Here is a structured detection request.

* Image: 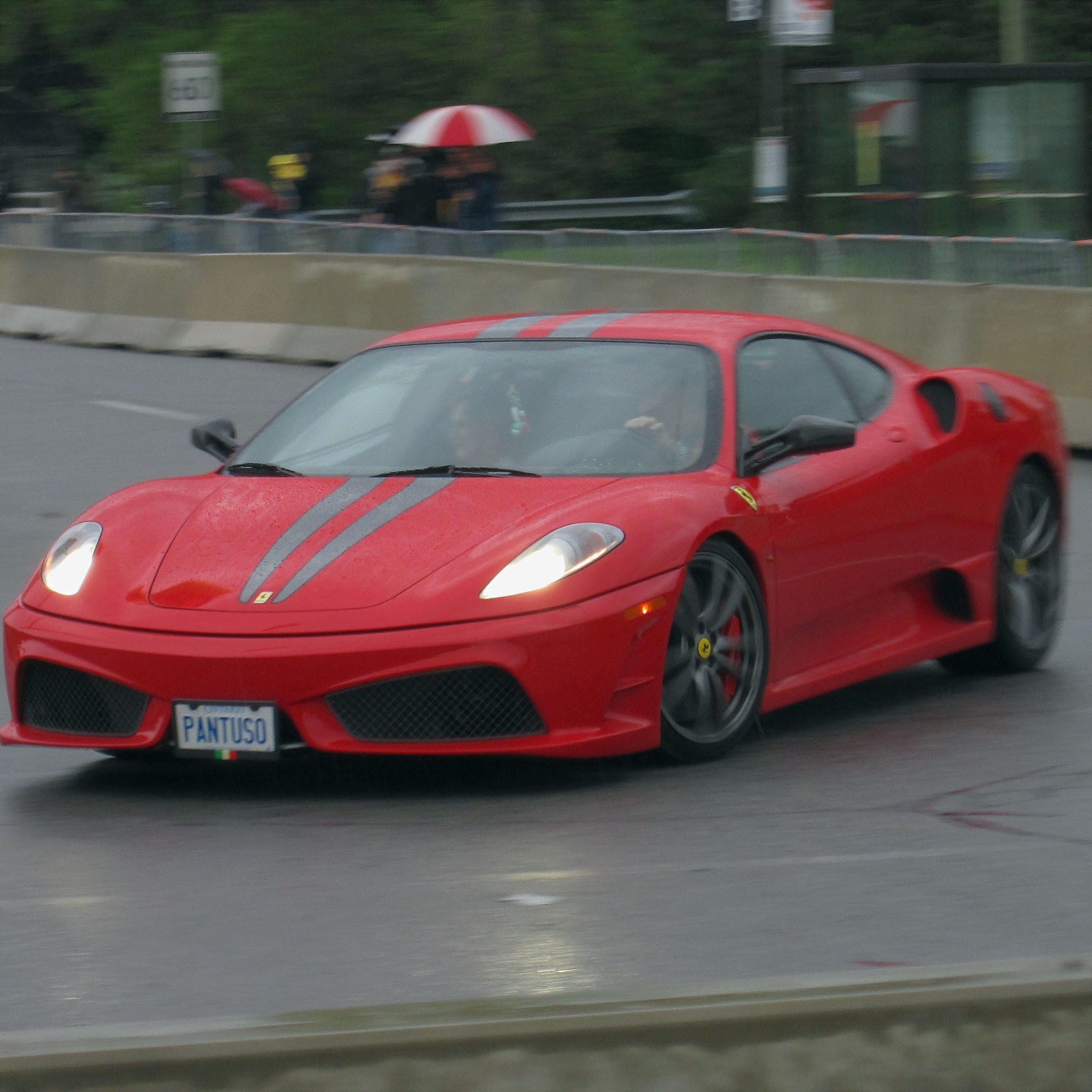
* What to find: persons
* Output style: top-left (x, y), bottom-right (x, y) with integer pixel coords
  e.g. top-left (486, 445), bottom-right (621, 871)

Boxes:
top-left (621, 342), bottom-right (761, 476)
top-left (261, 138), bottom-right (498, 234)
top-left (433, 394), bottom-right (494, 469)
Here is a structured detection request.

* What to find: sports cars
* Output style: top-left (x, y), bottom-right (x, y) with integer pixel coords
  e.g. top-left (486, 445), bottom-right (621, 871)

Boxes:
top-left (1, 309), bottom-right (1073, 772)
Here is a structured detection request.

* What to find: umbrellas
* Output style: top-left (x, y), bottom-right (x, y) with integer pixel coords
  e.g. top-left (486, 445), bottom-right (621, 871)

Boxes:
top-left (387, 104), bottom-right (535, 146)
